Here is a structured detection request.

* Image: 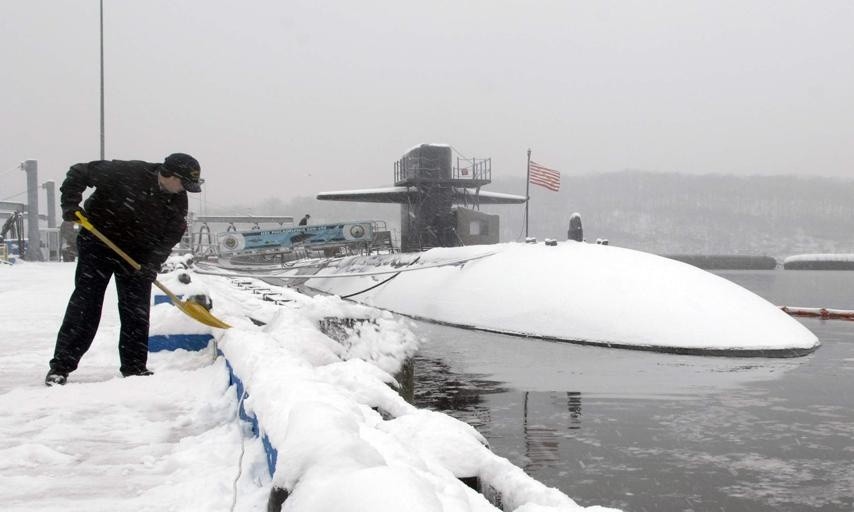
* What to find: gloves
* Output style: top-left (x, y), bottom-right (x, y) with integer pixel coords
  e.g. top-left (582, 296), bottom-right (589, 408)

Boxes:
top-left (134, 262), bottom-right (156, 283)
top-left (61, 202), bottom-right (85, 222)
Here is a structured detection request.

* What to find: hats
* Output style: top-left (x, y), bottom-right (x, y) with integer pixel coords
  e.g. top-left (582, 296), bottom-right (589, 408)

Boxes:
top-left (160, 154), bottom-right (203, 193)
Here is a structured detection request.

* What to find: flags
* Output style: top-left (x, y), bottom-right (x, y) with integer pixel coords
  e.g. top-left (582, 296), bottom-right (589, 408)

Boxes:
top-left (528, 160), bottom-right (560, 192)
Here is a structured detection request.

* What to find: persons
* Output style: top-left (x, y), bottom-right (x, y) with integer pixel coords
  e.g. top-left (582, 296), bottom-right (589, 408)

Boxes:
top-left (43, 152), bottom-right (202, 386)
top-left (298, 214), bottom-right (311, 231)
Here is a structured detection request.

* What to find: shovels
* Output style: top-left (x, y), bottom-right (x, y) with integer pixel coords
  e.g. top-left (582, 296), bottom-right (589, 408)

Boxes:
top-left (72, 212), bottom-right (232, 329)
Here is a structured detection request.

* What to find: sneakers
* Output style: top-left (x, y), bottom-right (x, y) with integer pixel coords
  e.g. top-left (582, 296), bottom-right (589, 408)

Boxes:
top-left (123, 368), bottom-right (153, 378)
top-left (44, 368), bottom-right (67, 385)
